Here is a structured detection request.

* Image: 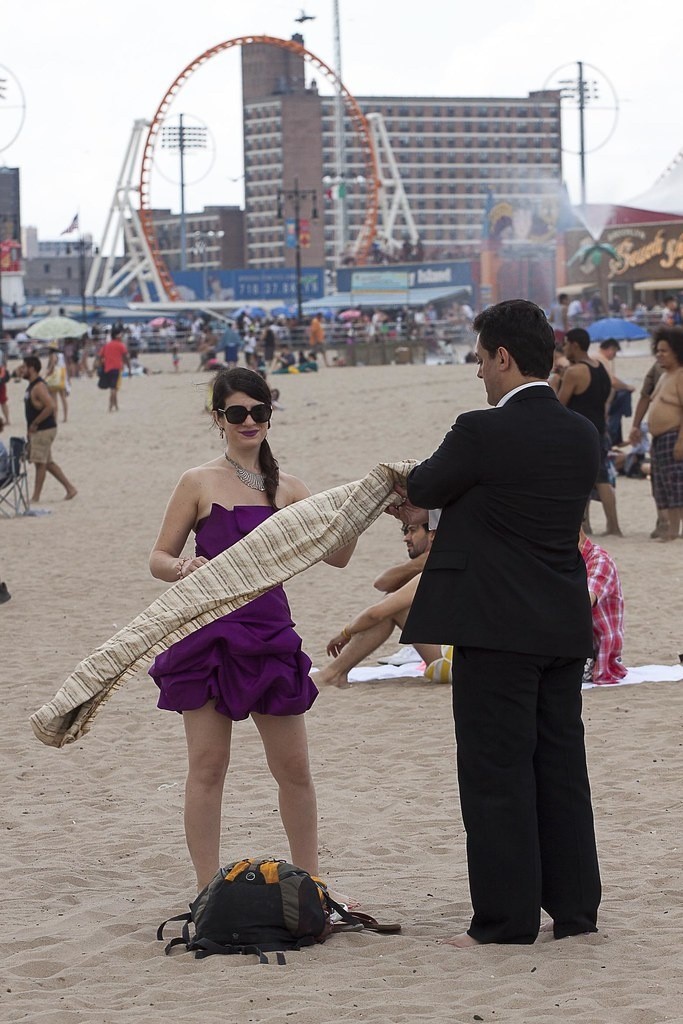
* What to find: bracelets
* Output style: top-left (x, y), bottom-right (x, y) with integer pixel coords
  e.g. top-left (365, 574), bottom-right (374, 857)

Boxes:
top-left (174, 556), bottom-right (189, 580)
top-left (341, 627), bottom-right (351, 639)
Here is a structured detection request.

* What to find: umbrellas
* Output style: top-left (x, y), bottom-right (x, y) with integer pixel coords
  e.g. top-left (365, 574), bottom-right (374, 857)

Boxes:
top-left (586, 317), bottom-right (650, 341)
top-left (26, 314), bottom-right (90, 340)
top-left (147, 317), bottom-right (175, 328)
top-left (230, 304), bottom-right (361, 322)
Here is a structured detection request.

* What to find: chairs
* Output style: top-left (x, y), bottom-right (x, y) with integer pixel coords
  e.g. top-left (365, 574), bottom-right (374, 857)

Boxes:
top-left (0, 437), bottom-right (33, 520)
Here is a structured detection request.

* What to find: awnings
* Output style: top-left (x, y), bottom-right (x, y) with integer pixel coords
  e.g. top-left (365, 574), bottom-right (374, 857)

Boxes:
top-left (294, 285), bottom-right (467, 307)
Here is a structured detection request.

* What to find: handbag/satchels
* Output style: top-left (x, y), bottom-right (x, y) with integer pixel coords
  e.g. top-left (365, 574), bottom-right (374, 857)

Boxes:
top-left (44, 365), bottom-right (64, 392)
top-left (97, 364), bottom-right (108, 389)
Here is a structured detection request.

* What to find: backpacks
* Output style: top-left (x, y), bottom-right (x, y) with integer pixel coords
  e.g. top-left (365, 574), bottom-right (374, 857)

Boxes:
top-left (156, 858), bottom-right (400, 962)
top-left (621, 450), bottom-right (647, 479)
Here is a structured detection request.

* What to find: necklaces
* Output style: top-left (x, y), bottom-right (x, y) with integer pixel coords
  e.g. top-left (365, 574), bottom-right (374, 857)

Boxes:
top-left (225, 452), bottom-right (266, 492)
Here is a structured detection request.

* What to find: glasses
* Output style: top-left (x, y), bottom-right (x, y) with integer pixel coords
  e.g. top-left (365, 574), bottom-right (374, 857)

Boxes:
top-left (218, 401), bottom-right (273, 425)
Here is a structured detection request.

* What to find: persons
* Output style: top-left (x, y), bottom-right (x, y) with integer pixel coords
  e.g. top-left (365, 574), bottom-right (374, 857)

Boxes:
top-left (11, 312), bottom-right (154, 380)
top-left (0, 328), bottom-right (133, 504)
top-left (309, 328), bottom-right (683, 689)
top-left (148, 367), bottom-right (359, 895)
top-left (548, 292), bottom-right (683, 339)
top-left (384, 298), bottom-right (602, 948)
top-left (171, 311), bottom-right (333, 374)
top-left (364, 303), bottom-right (438, 340)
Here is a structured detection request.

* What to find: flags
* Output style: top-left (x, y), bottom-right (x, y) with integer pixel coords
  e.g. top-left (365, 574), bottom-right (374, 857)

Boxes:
top-left (325, 181), bottom-right (345, 200)
top-left (59, 215), bottom-right (79, 235)
